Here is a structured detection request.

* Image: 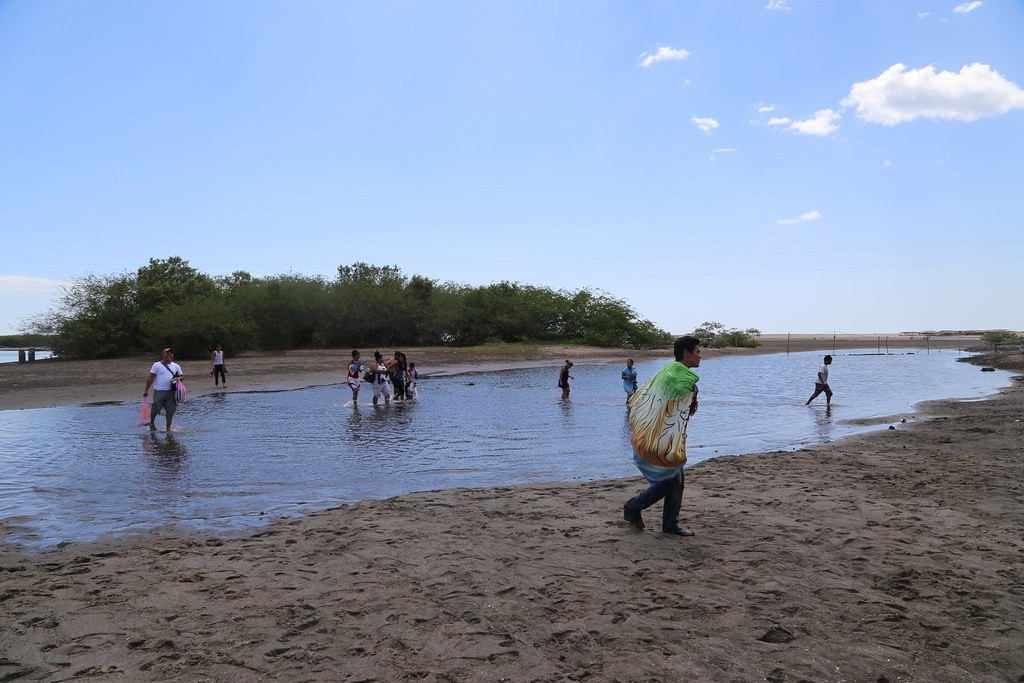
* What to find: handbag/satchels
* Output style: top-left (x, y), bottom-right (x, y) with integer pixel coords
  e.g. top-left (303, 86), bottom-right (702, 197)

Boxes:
top-left (137, 397), bottom-right (151, 427)
top-left (173, 378), bottom-right (186, 405)
top-left (404, 379), bottom-right (413, 398)
top-left (363, 371), bottom-right (375, 383)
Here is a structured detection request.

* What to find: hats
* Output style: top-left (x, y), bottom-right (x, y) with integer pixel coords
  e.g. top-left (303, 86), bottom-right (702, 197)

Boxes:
top-left (162, 348), bottom-right (174, 353)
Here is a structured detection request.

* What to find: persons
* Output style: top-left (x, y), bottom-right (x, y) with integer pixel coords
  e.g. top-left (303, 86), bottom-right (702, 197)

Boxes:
top-left (805, 355), bottom-right (833, 405)
top-left (143, 348), bottom-right (184, 431)
top-left (371, 351), bottom-right (418, 404)
top-left (211, 345), bottom-right (227, 388)
top-left (624, 336), bottom-right (702, 536)
top-left (622, 359), bottom-right (637, 404)
top-left (558, 360), bottom-right (574, 399)
top-left (347, 350), bottom-right (363, 408)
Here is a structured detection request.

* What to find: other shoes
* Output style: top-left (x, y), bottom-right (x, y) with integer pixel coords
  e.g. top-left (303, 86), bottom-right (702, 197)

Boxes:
top-left (215, 385), bottom-right (221, 389)
top-left (623, 511), bottom-right (645, 529)
top-left (223, 385), bottom-right (228, 388)
top-left (663, 526), bottom-right (694, 536)
